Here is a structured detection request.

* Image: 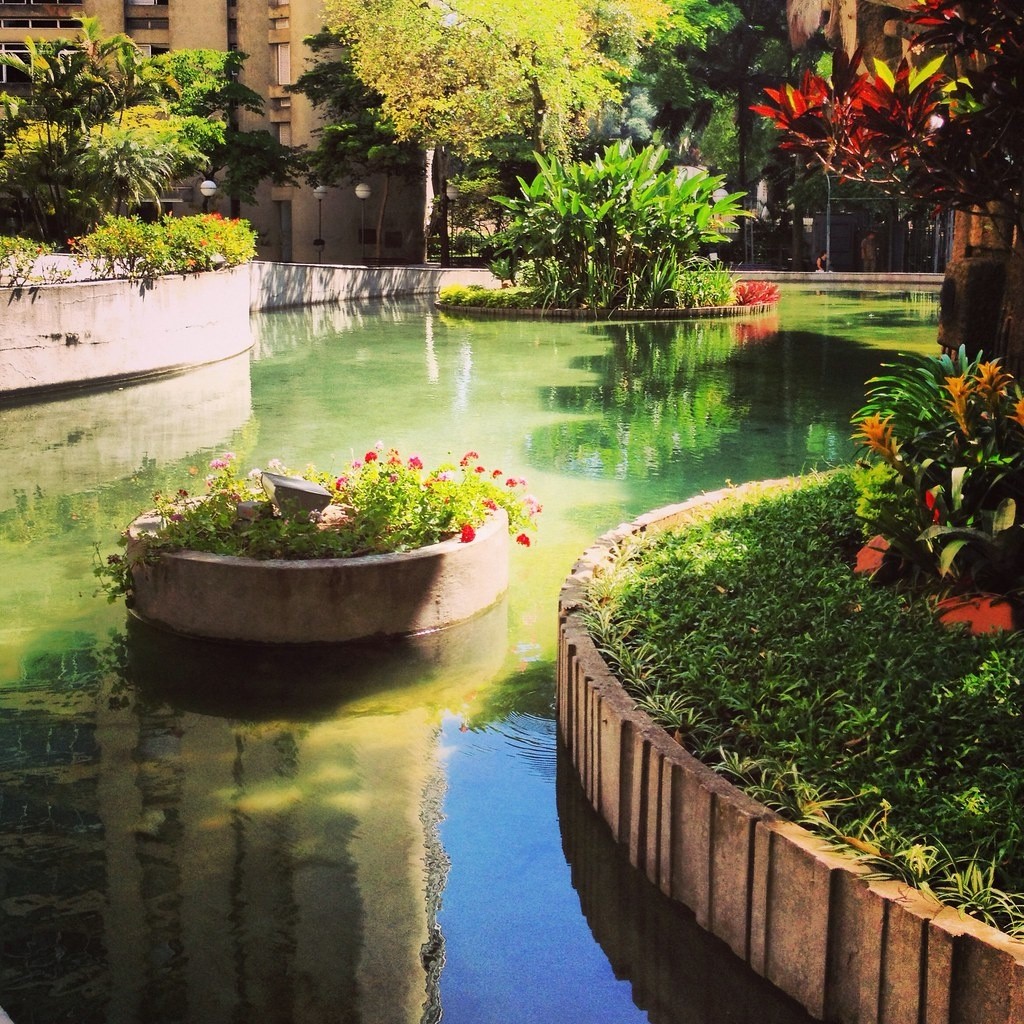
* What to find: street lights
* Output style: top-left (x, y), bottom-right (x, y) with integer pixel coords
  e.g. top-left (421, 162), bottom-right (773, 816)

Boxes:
top-left (446, 185), bottom-right (458, 268)
top-left (200, 180), bottom-right (217, 215)
top-left (313, 185), bottom-right (327, 265)
top-left (355, 182), bottom-right (373, 265)
top-left (712, 189), bottom-right (729, 271)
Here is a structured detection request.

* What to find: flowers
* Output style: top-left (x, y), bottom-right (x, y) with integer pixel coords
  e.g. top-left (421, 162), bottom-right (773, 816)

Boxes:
top-left (84, 453), bottom-right (562, 608)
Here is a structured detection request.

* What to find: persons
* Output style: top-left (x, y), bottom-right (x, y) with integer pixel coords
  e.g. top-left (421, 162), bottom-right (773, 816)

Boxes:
top-left (816, 250), bottom-right (827, 271)
top-left (861, 232), bottom-right (877, 272)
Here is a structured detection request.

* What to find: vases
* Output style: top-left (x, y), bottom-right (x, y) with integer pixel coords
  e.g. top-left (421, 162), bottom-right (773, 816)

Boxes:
top-left (131, 492), bottom-right (456, 644)
